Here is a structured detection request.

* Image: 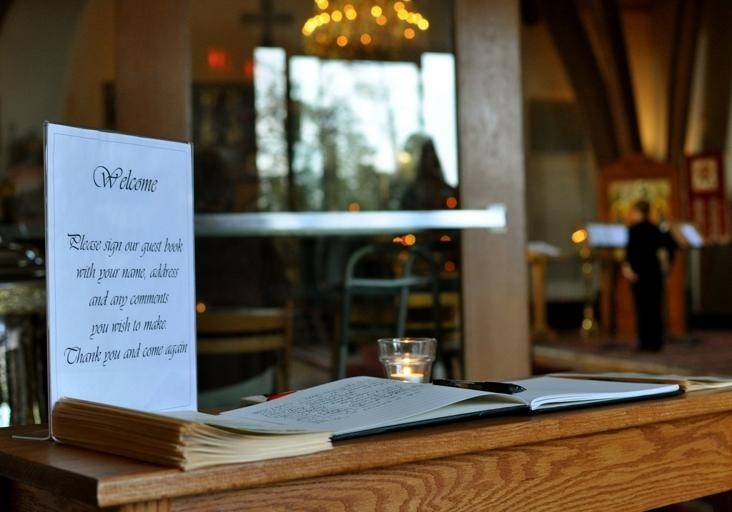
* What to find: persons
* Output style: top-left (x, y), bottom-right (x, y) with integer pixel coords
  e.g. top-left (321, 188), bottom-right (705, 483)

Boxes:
top-left (620, 198), bottom-right (680, 353)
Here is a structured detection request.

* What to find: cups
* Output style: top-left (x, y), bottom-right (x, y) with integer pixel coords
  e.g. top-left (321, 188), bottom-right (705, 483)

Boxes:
top-left (376, 338), bottom-right (438, 384)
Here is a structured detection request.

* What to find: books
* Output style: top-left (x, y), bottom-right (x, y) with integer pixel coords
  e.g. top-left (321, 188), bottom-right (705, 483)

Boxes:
top-left (53, 395), bottom-right (335, 471)
top-left (588, 372), bottom-right (732, 392)
top-left (223, 375), bottom-right (685, 442)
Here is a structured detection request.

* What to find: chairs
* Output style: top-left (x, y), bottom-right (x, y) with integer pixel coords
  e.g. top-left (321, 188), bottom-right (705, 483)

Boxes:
top-left (198, 296), bottom-right (295, 398)
top-left (335, 241), bottom-right (453, 382)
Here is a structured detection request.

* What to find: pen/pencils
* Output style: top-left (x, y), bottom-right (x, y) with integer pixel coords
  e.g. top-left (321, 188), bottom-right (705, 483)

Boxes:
top-left (433, 379), bottom-right (527, 393)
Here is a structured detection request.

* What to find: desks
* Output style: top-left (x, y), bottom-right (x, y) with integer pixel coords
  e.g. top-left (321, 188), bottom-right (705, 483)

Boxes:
top-left (0, 377), bottom-right (730, 512)
